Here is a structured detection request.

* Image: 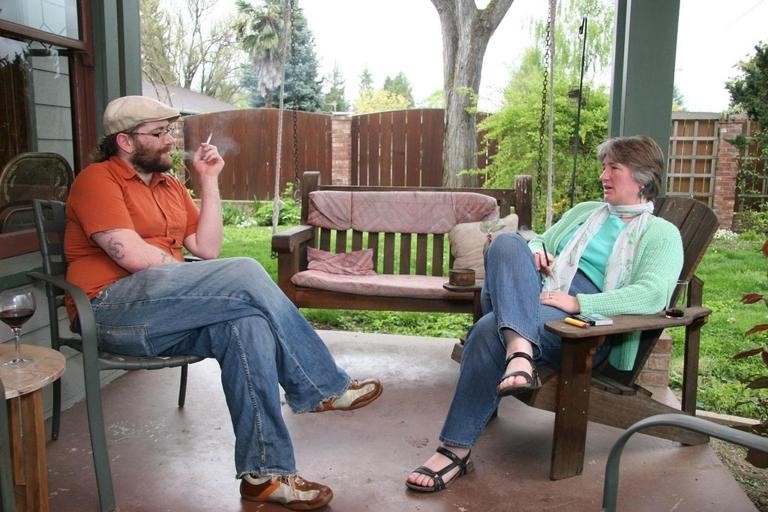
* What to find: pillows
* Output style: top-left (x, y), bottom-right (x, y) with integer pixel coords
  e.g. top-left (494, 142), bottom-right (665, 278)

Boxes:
top-left (303, 245), bottom-right (376, 275)
top-left (447, 209), bottom-right (521, 280)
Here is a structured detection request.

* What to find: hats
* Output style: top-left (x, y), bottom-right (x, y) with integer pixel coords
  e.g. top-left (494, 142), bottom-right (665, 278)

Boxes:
top-left (103, 94), bottom-right (181, 137)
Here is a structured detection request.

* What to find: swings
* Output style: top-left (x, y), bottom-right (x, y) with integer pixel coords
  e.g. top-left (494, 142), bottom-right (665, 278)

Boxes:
top-left (271, 1), bottom-right (555, 324)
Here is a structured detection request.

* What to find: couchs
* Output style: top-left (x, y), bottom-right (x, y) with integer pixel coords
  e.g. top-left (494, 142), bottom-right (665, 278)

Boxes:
top-left (270, 168), bottom-right (537, 329)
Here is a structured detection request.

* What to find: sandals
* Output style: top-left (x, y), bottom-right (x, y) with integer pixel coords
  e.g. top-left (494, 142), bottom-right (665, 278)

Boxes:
top-left (405, 446), bottom-right (473, 493)
top-left (498, 351), bottom-right (543, 398)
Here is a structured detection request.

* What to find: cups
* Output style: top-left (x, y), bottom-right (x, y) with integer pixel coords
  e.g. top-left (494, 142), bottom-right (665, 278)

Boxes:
top-left (665, 279), bottom-right (689, 320)
top-left (448, 268), bottom-right (476, 287)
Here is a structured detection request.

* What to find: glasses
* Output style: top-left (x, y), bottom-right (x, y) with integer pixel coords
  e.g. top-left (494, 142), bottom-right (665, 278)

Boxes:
top-left (124, 127), bottom-right (174, 140)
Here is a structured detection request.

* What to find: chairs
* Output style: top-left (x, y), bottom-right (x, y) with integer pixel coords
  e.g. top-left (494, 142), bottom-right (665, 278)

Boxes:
top-left (446, 196), bottom-right (723, 485)
top-left (2, 151), bottom-right (78, 236)
top-left (23, 195), bottom-right (213, 512)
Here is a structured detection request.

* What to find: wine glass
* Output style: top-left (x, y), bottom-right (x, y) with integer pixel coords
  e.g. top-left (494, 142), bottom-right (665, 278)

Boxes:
top-left (0, 291), bottom-right (38, 368)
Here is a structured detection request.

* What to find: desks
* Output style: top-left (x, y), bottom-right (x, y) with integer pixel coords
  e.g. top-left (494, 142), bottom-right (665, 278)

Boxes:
top-left (2, 340), bottom-right (69, 511)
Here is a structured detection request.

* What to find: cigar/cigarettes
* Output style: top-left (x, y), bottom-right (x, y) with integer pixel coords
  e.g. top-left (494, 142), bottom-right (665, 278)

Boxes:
top-left (207, 132), bottom-right (213, 145)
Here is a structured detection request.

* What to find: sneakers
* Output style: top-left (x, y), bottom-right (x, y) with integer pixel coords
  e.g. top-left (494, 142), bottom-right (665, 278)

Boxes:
top-left (307, 377), bottom-right (384, 412)
top-left (239, 469), bottom-right (333, 510)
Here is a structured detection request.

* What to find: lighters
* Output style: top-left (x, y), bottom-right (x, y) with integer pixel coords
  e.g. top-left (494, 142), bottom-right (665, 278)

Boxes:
top-left (563, 316), bottom-right (590, 328)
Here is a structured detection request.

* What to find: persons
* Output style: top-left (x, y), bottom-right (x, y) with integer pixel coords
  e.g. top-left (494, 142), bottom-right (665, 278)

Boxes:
top-left (405, 134), bottom-right (685, 492)
top-left (64, 94), bottom-right (382, 511)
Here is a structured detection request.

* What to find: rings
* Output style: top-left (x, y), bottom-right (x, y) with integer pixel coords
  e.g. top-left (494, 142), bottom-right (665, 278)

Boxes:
top-left (548, 295), bottom-right (552, 299)
